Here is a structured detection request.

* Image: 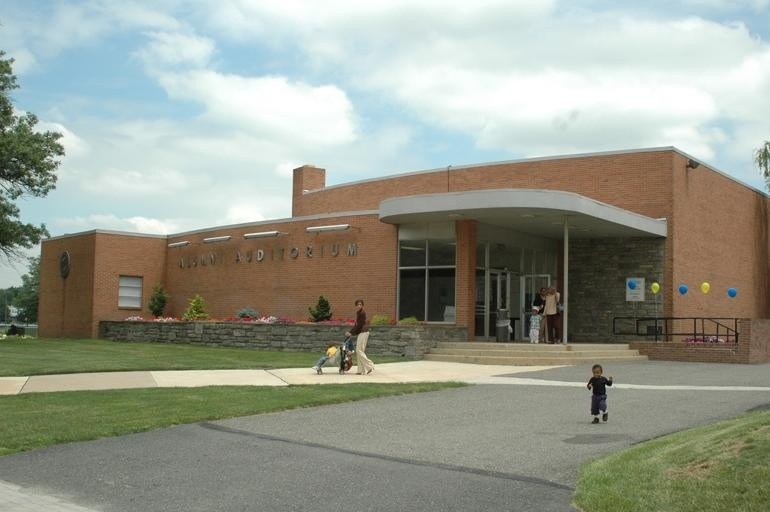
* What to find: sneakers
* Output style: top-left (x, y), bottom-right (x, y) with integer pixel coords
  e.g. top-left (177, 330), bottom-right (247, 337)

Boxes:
top-left (530, 339), bottom-right (560, 344)
top-left (592, 418), bottom-right (599, 424)
top-left (311, 366), bottom-right (321, 371)
top-left (603, 413), bottom-right (608, 421)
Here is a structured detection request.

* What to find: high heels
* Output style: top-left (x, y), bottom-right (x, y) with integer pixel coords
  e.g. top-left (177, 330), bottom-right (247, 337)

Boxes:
top-left (367, 367), bottom-right (374, 375)
top-left (356, 372), bottom-right (365, 375)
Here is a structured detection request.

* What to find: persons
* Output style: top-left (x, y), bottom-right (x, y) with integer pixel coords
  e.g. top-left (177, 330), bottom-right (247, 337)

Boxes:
top-left (345, 299), bottom-right (375, 375)
top-left (312, 341), bottom-right (337, 371)
top-left (529, 286), bottom-right (561, 344)
top-left (587, 365), bottom-right (612, 423)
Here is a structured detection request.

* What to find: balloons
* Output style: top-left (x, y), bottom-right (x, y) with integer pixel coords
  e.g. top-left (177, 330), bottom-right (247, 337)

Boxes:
top-left (678, 284), bottom-right (688, 296)
top-left (650, 281), bottom-right (660, 294)
top-left (627, 279), bottom-right (637, 289)
top-left (728, 287), bottom-right (737, 298)
top-left (701, 282), bottom-right (711, 294)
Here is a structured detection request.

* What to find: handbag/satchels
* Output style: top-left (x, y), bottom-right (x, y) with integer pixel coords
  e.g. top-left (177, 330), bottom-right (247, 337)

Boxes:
top-left (340, 346), bottom-right (352, 371)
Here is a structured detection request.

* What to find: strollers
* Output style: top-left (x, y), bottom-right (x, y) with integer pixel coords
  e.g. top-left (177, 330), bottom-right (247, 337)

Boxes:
top-left (316, 337), bottom-right (351, 375)
